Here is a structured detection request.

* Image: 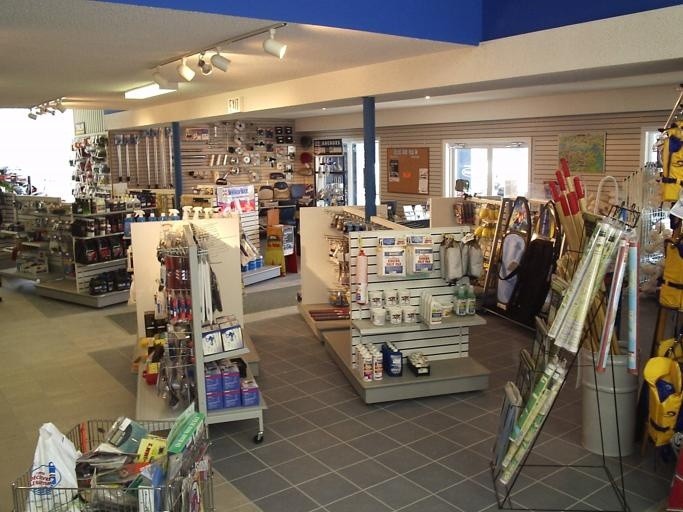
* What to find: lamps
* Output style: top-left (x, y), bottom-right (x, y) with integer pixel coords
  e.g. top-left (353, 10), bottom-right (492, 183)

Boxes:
top-left (179, 28), bottom-right (288, 83)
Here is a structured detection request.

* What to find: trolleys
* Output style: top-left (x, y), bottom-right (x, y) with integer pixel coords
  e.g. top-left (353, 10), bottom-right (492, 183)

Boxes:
top-left (10, 418), bottom-right (214, 512)
top-left (130, 218), bottom-right (268, 444)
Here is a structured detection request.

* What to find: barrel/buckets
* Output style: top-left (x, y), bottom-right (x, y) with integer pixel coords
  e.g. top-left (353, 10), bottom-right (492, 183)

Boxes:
top-left (78, 238), bottom-right (132, 295)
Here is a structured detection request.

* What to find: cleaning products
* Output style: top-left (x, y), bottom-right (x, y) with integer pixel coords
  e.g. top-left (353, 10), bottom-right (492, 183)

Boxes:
top-left (122, 205), bottom-right (232, 237)
